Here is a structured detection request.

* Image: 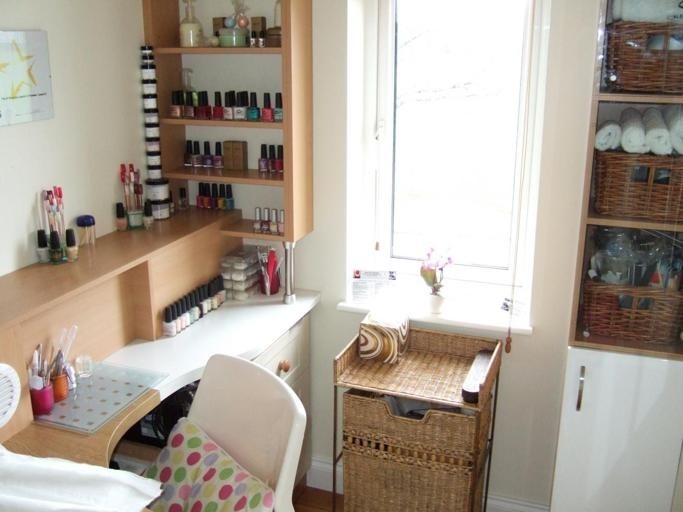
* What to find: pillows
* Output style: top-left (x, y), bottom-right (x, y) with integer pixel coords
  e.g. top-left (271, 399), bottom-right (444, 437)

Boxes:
top-left (142, 417), bottom-right (275, 512)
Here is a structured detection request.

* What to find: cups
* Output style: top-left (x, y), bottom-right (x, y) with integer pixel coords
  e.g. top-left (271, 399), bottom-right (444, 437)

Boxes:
top-left (75, 356), bottom-right (93, 378)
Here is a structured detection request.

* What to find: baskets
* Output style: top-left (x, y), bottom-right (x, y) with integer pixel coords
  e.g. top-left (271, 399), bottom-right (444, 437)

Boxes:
top-left (583, 282), bottom-right (683, 345)
top-left (594, 150), bottom-right (683, 222)
top-left (606, 20), bottom-right (683, 93)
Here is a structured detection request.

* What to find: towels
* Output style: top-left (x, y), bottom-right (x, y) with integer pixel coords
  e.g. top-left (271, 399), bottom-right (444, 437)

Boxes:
top-left (596, 104), bottom-right (683, 155)
top-left (611, 0), bottom-right (683, 24)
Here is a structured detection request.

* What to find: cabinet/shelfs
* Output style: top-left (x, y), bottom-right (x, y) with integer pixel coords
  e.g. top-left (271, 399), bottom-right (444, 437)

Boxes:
top-left (332, 327), bottom-right (502, 512)
top-left (143, 0), bottom-right (313, 243)
top-left (549, 0), bottom-right (683, 512)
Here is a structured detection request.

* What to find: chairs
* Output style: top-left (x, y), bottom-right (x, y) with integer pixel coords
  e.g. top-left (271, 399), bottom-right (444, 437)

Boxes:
top-left (187, 353), bottom-right (308, 512)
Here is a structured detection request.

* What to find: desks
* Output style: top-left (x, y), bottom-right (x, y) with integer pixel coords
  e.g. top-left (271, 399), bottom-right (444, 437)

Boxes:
top-left (0, 210), bottom-right (323, 512)
